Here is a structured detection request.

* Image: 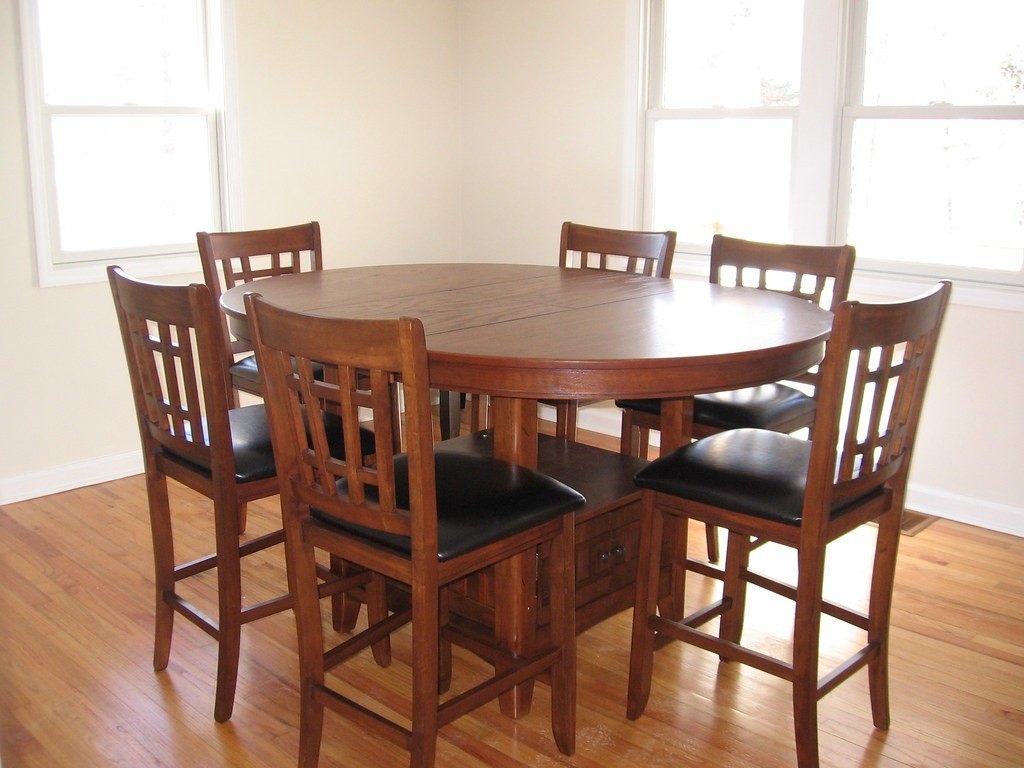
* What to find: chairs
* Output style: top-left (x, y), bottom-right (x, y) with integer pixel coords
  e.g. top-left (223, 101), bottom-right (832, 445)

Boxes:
top-left (106, 264), bottom-right (394, 724)
top-left (624, 280), bottom-right (957, 767)
top-left (616, 230), bottom-right (857, 562)
top-left (486, 221), bottom-right (679, 460)
top-left (241, 289), bottom-right (588, 767)
top-left (195, 220), bottom-right (400, 535)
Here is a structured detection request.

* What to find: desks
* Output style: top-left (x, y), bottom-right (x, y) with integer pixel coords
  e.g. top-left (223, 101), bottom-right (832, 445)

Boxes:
top-left (220, 261), bottom-right (835, 719)
